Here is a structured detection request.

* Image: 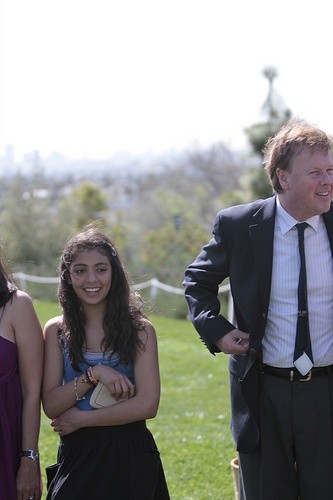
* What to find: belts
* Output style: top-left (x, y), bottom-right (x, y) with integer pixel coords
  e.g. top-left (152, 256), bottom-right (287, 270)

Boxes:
top-left (261, 368), bottom-right (333, 381)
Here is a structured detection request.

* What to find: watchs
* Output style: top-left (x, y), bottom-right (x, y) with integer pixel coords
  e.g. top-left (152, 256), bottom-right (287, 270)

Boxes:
top-left (20, 449), bottom-right (39, 461)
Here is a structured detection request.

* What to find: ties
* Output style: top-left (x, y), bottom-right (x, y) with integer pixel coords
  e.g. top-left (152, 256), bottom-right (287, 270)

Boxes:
top-left (292, 223), bottom-right (314, 374)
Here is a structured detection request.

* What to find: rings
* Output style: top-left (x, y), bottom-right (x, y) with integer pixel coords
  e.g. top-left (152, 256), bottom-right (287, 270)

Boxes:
top-left (30, 497), bottom-right (33, 499)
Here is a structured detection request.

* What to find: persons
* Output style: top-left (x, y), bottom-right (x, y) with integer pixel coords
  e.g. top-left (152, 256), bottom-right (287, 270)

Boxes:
top-left (182, 124), bottom-right (333, 500)
top-left (44, 228), bottom-right (171, 500)
top-left (0, 264), bottom-right (43, 500)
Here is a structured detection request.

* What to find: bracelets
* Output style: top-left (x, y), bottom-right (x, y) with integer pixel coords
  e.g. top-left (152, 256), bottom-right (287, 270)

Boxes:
top-left (73, 376), bottom-right (86, 401)
top-left (81, 365), bottom-right (98, 388)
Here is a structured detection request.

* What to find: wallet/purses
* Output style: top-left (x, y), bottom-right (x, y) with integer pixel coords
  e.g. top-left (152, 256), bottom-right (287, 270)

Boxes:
top-left (88, 382), bottom-right (127, 408)
